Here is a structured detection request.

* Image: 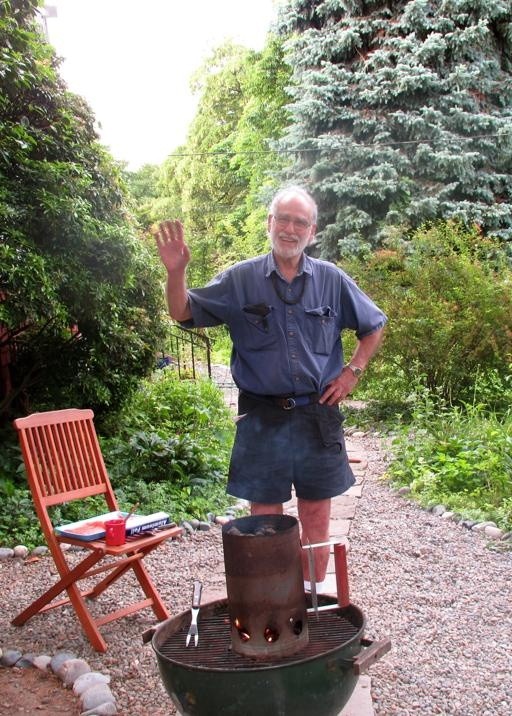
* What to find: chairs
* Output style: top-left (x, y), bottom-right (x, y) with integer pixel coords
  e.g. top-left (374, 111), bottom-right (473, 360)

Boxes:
top-left (9, 405), bottom-right (184, 653)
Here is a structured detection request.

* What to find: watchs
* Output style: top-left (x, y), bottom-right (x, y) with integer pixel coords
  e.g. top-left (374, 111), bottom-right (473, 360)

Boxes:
top-left (345, 363), bottom-right (363, 379)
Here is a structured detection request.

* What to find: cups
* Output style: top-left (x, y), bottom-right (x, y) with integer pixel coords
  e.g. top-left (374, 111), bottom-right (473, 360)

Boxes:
top-left (104, 519), bottom-right (126, 547)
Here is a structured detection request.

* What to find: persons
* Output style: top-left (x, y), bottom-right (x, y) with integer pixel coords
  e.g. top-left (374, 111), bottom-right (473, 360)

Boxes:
top-left (152, 183), bottom-right (388, 597)
